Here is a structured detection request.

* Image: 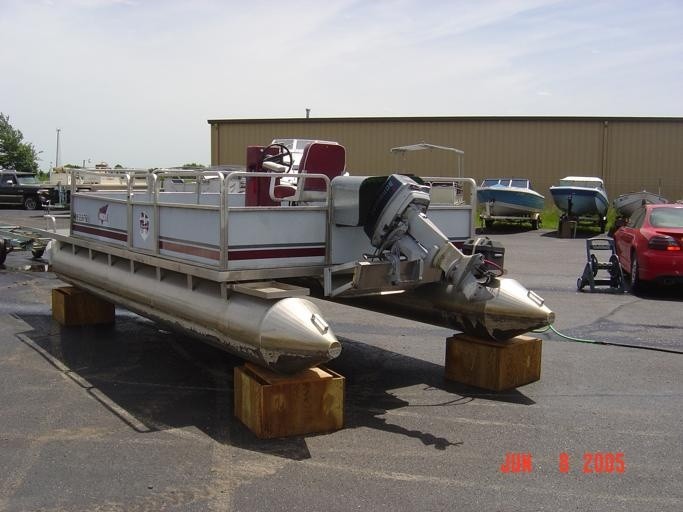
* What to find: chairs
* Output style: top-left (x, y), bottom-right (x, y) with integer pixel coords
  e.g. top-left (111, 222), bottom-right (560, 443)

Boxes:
top-left (270, 142), bottom-right (346, 201)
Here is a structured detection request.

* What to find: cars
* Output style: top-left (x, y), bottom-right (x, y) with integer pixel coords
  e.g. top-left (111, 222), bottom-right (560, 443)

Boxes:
top-left (611, 202), bottom-right (683, 290)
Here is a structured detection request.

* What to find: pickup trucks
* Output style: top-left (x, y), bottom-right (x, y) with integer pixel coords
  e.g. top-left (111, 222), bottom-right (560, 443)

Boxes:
top-left (0, 168), bottom-right (78, 211)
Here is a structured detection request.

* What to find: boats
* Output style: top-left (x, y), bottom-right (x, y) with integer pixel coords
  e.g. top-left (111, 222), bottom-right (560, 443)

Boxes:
top-left (548, 175), bottom-right (609, 219)
top-left (477, 178), bottom-right (545, 216)
top-left (612, 189), bottom-right (669, 217)
